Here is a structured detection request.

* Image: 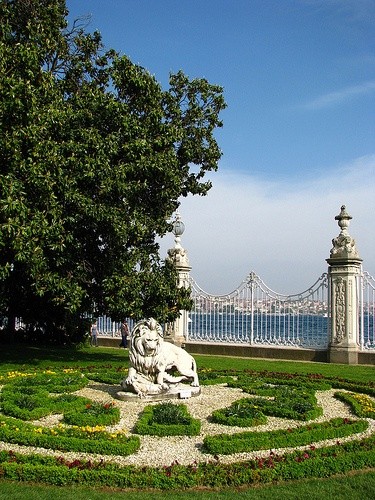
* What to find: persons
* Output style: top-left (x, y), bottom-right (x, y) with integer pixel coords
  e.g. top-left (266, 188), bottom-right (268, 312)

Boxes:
top-left (89, 321), bottom-right (101, 347)
top-left (119, 321), bottom-right (130, 349)
